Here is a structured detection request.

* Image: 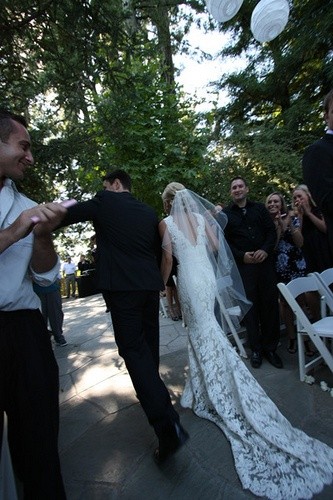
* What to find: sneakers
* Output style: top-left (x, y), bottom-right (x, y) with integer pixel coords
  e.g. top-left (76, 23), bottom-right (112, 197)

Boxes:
top-left (55, 335), bottom-right (67, 346)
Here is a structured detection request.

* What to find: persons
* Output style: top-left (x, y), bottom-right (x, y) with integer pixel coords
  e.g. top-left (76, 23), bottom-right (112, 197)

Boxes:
top-left (33, 281), bottom-right (67, 347)
top-left (202, 202), bottom-right (226, 222)
top-left (216, 177), bottom-right (284, 369)
top-left (292, 184), bottom-right (333, 341)
top-left (51, 169), bottom-right (190, 462)
top-left (0, 108), bottom-right (66, 500)
top-left (165, 257), bottom-right (183, 321)
top-left (78, 255), bottom-right (91, 275)
top-left (159, 182), bottom-right (250, 412)
top-left (264, 191), bottom-right (315, 356)
top-left (303, 91), bottom-right (333, 235)
top-left (62, 256), bottom-right (78, 300)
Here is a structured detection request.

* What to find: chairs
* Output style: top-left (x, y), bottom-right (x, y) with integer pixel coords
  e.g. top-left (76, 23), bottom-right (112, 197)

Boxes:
top-left (215, 268), bottom-right (333, 386)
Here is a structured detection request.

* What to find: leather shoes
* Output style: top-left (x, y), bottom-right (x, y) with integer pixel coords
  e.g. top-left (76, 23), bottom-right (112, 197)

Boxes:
top-left (269, 352), bottom-right (282, 368)
top-left (154, 421), bottom-right (189, 461)
top-left (251, 351), bottom-right (262, 368)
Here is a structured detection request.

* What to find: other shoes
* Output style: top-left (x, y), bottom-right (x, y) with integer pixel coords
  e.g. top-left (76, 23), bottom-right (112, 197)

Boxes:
top-left (172, 315), bottom-right (178, 321)
top-left (288, 337), bottom-right (297, 353)
top-left (304, 339), bottom-right (314, 356)
top-left (178, 311), bottom-right (182, 319)
